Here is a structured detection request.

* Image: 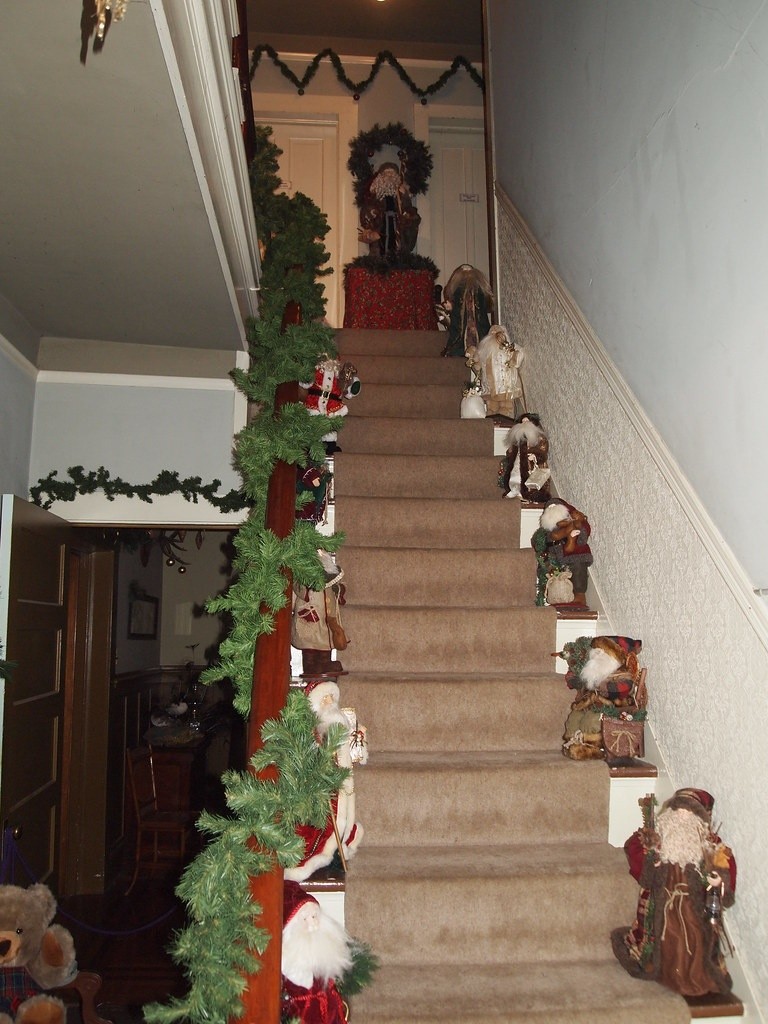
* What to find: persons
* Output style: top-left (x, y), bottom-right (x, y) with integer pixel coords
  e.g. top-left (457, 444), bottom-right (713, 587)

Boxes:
top-left (289, 545), bottom-right (346, 673)
top-left (297, 349), bottom-right (357, 454)
top-left (614, 787), bottom-right (737, 1001)
top-left (295, 456), bottom-right (332, 531)
top-left (359, 161), bottom-right (421, 259)
top-left (440, 264), bottom-right (492, 356)
top-left (551, 635), bottom-right (646, 760)
top-left (498, 412), bottom-right (552, 501)
top-left (279, 879), bottom-right (356, 1024)
top-left (531, 498), bottom-right (593, 608)
top-left (467, 324), bottom-right (525, 419)
top-left (284, 676), bottom-right (369, 884)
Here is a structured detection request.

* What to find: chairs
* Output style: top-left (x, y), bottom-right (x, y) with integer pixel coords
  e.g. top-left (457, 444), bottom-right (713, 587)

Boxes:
top-left (124, 744), bottom-right (206, 897)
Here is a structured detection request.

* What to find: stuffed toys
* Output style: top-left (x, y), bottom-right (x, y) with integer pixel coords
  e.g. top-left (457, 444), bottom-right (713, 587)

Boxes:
top-left (0, 882), bottom-right (77, 1024)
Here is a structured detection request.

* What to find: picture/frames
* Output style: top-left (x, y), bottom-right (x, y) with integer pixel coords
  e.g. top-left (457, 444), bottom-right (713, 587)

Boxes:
top-left (129, 595), bottom-right (160, 641)
